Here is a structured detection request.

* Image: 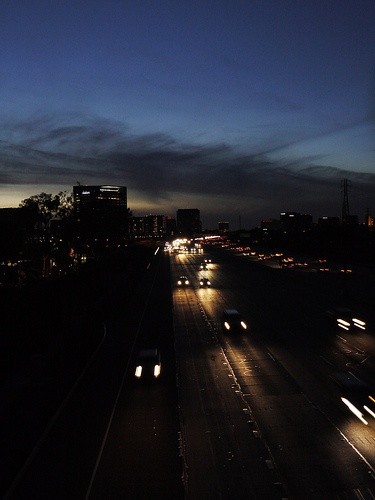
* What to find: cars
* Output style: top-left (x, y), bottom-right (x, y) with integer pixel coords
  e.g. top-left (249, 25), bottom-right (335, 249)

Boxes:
top-left (176, 275), bottom-right (191, 287)
top-left (221, 308), bottom-right (248, 336)
top-left (131, 348), bottom-right (161, 383)
top-left (160, 234), bottom-right (354, 273)
top-left (327, 307), bottom-right (365, 335)
top-left (200, 279), bottom-right (212, 288)
